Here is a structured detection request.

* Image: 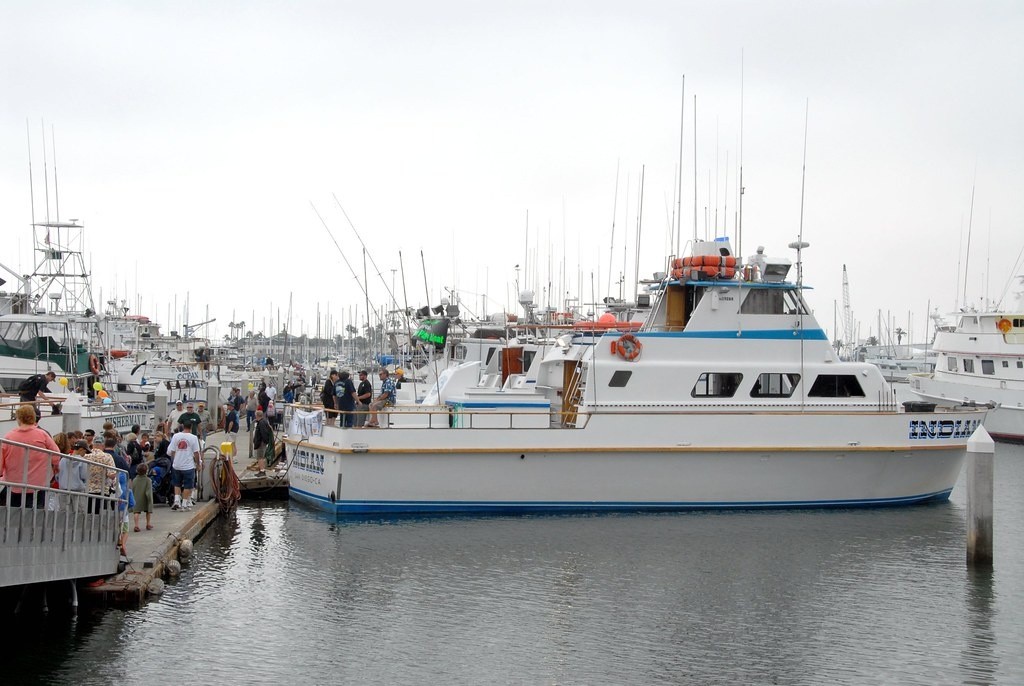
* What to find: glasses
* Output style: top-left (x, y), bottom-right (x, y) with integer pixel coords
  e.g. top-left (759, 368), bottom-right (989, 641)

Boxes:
top-left (85, 434), bottom-right (91, 436)
top-left (187, 407), bottom-right (192, 408)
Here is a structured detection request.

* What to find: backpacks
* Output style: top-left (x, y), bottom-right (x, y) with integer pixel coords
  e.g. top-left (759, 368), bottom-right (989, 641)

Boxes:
top-left (18, 374), bottom-right (40, 394)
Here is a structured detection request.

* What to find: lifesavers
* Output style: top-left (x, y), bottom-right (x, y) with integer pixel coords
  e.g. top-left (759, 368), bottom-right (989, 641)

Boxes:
top-left (90, 353), bottom-right (100, 375)
top-left (998, 318), bottom-right (1012, 333)
top-left (617, 334), bottom-right (641, 360)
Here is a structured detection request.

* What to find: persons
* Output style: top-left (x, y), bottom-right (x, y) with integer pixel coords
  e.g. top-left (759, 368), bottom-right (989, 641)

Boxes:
top-left (224, 401), bottom-right (239, 464)
top-left (33, 400), bottom-right (210, 556)
top-left (0, 404), bottom-right (61, 508)
top-left (394, 369), bottom-right (407, 389)
top-left (227, 359), bottom-right (321, 431)
top-left (132, 463), bottom-right (154, 532)
top-left (356, 370), bottom-right (372, 428)
top-left (367, 368), bottom-right (396, 427)
top-left (252, 409), bottom-right (274, 477)
top-left (266, 357), bottom-right (274, 366)
top-left (17, 372), bottom-right (57, 423)
top-left (319, 369), bottom-right (339, 425)
top-left (332, 371), bottom-right (362, 428)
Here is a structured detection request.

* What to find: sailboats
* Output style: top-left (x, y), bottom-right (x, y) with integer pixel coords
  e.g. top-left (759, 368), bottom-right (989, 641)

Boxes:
top-left (0, 54), bottom-right (991, 520)
top-left (912, 165), bottom-right (1024, 444)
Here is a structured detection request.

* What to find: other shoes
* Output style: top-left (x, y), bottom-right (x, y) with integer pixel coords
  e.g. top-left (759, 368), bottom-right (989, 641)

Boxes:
top-left (191, 497), bottom-right (196, 504)
top-left (146, 525), bottom-right (153, 530)
top-left (171, 501), bottom-right (179, 510)
top-left (368, 421), bottom-right (379, 426)
top-left (121, 545), bottom-right (127, 556)
top-left (180, 507), bottom-right (191, 512)
top-left (134, 527), bottom-right (141, 531)
top-left (254, 471), bottom-right (266, 477)
top-left (186, 501), bottom-right (193, 506)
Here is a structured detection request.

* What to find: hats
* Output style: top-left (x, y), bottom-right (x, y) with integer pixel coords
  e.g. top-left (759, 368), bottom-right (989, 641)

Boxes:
top-left (224, 401), bottom-right (235, 406)
top-left (35, 409), bottom-right (41, 417)
top-left (198, 402), bottom-right (205, 407)
top-left (47, 372), bottom-right (57, 382)
top-left (73, 440), bottom-right (92, 454)
top-left (151, 431), bottom-right (162, 436)
top-left (331, 370), bottom-right (338, 374)
top-left (397, 369), bottom-right (403, 375)
top-left (358, 371), bottom-right (367, 376)
top-left (176, 400), bottom-right (182, 404)
